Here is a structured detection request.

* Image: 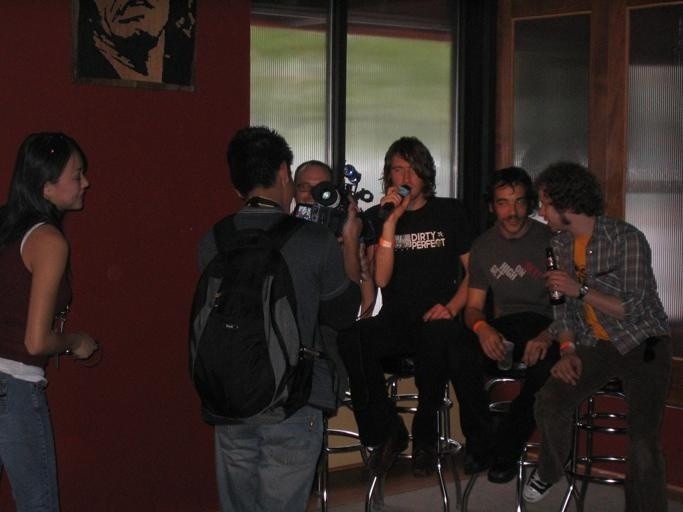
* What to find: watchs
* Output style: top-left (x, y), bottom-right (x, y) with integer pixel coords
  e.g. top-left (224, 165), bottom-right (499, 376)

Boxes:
top-left (576, 281), bottom-right (590, 300)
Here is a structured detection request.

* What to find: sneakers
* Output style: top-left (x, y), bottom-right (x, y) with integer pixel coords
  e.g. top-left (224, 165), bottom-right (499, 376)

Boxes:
top-left (408, 438), bottom-right (437, 478)
top-left (485, 441), bottom-right (523, 483)
top-left (454, 438), bottom-right (484, 476)
top-left (521, 465), bottom-right (554, 505)
top-left (372, 435), bottom-right (406, 474)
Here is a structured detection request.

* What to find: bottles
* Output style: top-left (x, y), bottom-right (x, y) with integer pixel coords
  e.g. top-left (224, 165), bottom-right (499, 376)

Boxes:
top-left (546, 247), bottom-right (566, 305)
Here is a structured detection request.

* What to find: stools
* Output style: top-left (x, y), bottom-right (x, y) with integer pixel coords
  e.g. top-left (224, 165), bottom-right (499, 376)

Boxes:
top-left (551, 378), bottom-right (643, 510)
top-left (465, 374), bottom-right (581, 509)
top-left (365, 361), bottom-right (463, 509)
top-left (316, 371), bottom-right (386, 512)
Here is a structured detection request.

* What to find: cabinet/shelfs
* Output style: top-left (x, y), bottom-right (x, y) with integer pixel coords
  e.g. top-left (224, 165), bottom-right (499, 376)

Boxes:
top-left (574, 359), bottom-right (682, 501)
top-left (494, 1), bottom-right (682, 356)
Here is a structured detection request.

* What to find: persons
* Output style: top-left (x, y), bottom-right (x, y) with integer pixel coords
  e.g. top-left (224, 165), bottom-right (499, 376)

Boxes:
top-left (0, 131), bottom-right (98, 512)
top-left (443, 162), bottom-right (561, 484)
top-left (336, 135), bottom-right (477, 480)
top-left (196, 126), bottom-right (363, 512)
top-left (293, 160), bottom-right (376, 407)
top-left (522, 160), bottom-right (675, 512)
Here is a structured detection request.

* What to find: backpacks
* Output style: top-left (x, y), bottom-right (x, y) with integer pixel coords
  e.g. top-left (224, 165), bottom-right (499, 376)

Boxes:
top-left (186, 212), bottom-right (327, 427)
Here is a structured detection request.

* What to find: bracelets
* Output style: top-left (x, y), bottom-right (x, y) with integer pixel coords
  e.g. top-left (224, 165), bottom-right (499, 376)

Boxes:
top-left (379, 238), bottom-right (396, 249)
top-left (63, 335), bottom-right (71, 354)
top-left (472, 319), bottom-right (486, 332)
top-left (559, 341), bottom-right (577, 353)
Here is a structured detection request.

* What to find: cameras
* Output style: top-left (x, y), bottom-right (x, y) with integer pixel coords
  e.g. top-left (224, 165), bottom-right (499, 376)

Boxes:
top-left (291, 201), bottom-right (368, 237)
top-left (309, 164), bottom-right (361, 208)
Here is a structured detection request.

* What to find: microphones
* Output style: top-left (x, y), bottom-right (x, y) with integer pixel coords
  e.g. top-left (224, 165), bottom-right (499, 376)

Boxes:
top-left (376, 185), bottom-right (411, 220)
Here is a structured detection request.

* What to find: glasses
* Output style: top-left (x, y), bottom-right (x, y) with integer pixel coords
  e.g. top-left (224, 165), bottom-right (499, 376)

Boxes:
top-left (293, 182), bottom-right (313, 194)
